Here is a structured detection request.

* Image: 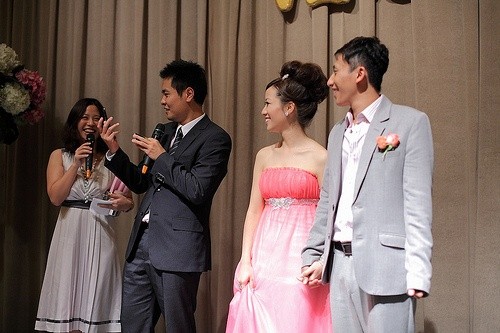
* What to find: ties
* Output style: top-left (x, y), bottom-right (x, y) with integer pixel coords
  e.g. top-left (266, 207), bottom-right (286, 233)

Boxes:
top-left (169, 126), bottom-right (183, 156)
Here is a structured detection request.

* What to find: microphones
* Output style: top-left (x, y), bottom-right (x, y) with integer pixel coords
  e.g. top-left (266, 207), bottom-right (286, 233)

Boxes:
top-left (86, 134), bottom-right (96, 178)
top-left (142, 123), bottom-right (165, 174)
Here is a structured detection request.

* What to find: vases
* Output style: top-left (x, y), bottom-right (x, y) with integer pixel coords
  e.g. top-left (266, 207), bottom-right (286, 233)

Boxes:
top-left (0, 108), bottom-right (19, 145)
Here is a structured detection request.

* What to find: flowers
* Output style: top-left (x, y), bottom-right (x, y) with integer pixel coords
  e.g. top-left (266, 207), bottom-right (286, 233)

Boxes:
top-left (0, 42), bottom-right (46, 124)
top-left (375, 131), bottom-right (401, 161)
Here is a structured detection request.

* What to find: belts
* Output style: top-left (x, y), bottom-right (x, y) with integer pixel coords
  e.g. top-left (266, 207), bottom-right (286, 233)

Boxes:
top-left (330, 241), bottom-right (352, 254)
top-left (62, 200), bottom-right (92, 209)
top-left (141, 222), bottom-right (149, 230)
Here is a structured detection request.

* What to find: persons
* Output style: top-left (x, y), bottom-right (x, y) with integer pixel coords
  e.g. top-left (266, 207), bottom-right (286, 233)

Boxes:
top-left (233, 60), bottom-right (329, 332)
top-left (97, 59), bottom-right (232, 333)
top-left (47, 98), bottom-right (134, 333)
top-left (301, 36), bottom-right (433, 333)
